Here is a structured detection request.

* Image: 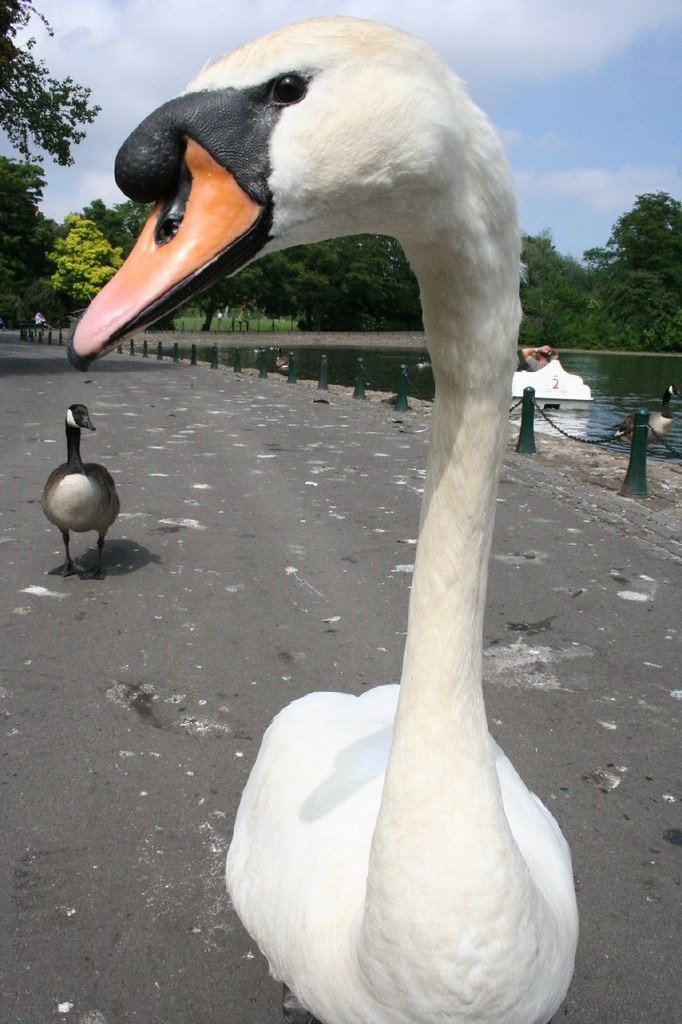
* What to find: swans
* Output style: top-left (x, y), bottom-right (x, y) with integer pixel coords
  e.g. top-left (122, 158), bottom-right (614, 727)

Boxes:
top-left (71, 16), bottom-right (580, 1024)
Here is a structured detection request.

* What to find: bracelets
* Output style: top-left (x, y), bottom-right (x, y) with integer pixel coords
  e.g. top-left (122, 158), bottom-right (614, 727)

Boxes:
top-left (535, 348), bottom-right (538, 352)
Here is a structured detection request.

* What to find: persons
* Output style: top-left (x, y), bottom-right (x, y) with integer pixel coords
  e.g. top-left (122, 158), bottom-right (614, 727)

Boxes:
top-left (246, 300), bottom-right (256, 318)
top-left (234, 308), bottom-right (245, 321)
top-left (0, 318), bottom-right (6, 331)
top-left (35, 312), bottom-right (46, 327)
top-left (521, 346), bottom-right (558, 372)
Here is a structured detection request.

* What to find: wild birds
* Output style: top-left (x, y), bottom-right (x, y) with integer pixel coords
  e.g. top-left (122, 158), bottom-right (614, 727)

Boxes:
top-left (42, 401), bottom-right (122, 582)
top-left (275, 347), bottom-right (289, 369)
top-left (612, 384), bottom-right (682, 448)
